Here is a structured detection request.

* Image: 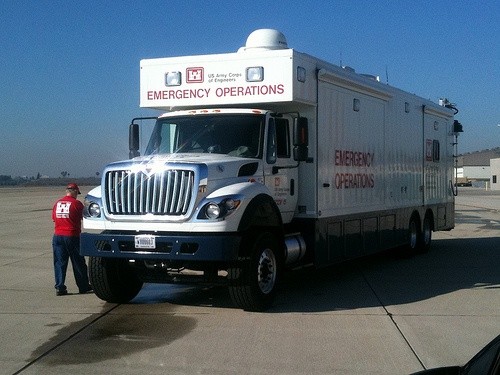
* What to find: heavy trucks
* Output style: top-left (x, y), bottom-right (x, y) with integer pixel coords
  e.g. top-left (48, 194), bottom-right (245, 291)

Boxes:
top-left (78, 28), bottom-right (465, 309)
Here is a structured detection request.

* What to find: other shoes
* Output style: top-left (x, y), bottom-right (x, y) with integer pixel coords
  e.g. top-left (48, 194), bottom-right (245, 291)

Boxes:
top-left (86, 284), bottom-right (93, 292)
top-left (56, 289), bottom-right (67, 296)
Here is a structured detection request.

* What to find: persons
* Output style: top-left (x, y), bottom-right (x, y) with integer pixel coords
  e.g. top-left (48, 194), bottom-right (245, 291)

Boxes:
top-left (51, 182), bottom-right (93, 296)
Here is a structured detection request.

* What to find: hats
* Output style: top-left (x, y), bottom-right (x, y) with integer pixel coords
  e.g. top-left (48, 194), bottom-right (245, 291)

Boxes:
top-left (67, 183), bottom-right (81, 194)
